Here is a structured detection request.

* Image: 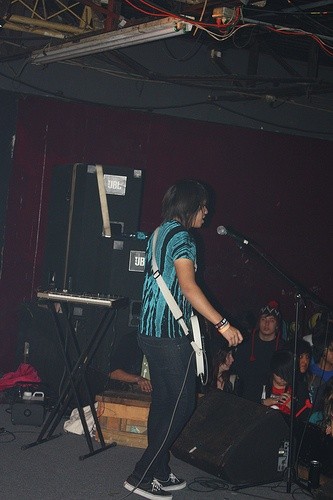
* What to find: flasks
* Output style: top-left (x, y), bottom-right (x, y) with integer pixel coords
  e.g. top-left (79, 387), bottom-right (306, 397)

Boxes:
top-left (307, 460), bottom-right (320, 489)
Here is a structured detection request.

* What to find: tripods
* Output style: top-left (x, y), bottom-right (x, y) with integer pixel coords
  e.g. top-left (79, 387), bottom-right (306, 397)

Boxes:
top-left (232, 244), bottom-right (322, 496)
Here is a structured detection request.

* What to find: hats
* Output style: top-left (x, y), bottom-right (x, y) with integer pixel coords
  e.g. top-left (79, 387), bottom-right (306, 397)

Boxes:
top-left (270, 352), bottom-right (298, 382)
top-left (297, 339), bottom-right (312, 357)
top-left (259, 299), bottom-right (281, 322)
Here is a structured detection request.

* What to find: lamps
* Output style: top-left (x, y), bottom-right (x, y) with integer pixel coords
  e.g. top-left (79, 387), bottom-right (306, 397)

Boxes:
top-left (30, 15), bottom-right (195, 66)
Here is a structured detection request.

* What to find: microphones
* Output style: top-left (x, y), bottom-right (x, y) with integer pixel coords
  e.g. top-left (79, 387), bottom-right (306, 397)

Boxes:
top-left (216, 226), bottom-right (249, 245)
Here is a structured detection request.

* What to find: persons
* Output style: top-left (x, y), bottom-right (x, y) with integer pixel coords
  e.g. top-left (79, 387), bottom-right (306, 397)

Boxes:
top-left (125, 178), bottom-right (244, 500)
top-left (104, 301), bottom-right (333, 438)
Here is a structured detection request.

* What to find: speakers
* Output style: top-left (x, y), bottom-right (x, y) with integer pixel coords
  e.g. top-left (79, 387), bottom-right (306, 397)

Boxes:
top-left (170, 391), bottom-right (291, 485)
top-left (21, 163), bottom-right (151, 407)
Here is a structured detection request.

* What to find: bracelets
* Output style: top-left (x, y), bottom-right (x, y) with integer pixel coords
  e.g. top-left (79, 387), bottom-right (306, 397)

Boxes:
top-left (219, 323), bottom-right (230, 333)
top-left (215, 318), bottom-right (226, 329)
top-left (218, 321), bottom-right (228, 329)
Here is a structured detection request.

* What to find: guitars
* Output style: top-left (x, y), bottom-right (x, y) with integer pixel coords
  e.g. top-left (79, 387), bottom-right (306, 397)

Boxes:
top-left (190, 313), bottom-right (213, 385)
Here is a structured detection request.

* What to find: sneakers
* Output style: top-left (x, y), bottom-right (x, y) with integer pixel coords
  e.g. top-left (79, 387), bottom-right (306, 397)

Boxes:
top-left (122, 474), bottom-right (172, 500)
top-left (153, 472), bottom-right (187, 491)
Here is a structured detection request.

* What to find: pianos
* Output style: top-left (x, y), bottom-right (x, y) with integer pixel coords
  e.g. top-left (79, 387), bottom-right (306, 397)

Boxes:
top-left (23, 290), bottom-right (131, 461)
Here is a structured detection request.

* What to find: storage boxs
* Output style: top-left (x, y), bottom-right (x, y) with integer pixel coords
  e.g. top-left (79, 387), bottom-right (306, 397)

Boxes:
top-left (94, 390), bottom-right (152, 449)
